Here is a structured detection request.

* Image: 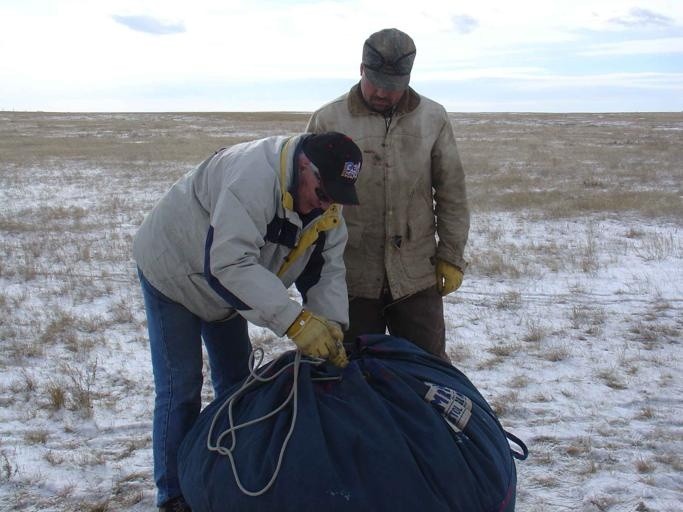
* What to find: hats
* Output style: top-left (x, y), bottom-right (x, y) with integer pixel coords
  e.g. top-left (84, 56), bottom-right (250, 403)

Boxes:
top-left (363, 29), bottom-right (417, 92)
top-left (303, 130), bottom-right (362, 206)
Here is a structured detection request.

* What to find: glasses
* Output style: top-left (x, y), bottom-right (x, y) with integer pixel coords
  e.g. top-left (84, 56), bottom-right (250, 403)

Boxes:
top-left (313, 187), bottom-right (328, 202)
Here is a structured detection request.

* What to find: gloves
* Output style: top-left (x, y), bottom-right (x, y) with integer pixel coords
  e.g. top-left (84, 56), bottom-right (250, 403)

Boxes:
top-left (436, 260), bottom-right (464, 297)
top-left (287, 308), bottom-right (342, 359)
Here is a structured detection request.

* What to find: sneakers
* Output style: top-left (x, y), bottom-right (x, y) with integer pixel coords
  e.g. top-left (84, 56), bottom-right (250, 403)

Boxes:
top-left (164, 496), bottom-right (190, 512)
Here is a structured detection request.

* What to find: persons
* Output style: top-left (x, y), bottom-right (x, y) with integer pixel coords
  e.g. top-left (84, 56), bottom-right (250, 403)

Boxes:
top-left (131, 132), bottom-right (363, 511)
top-left (305, 28), bottom-right (472, 362)
